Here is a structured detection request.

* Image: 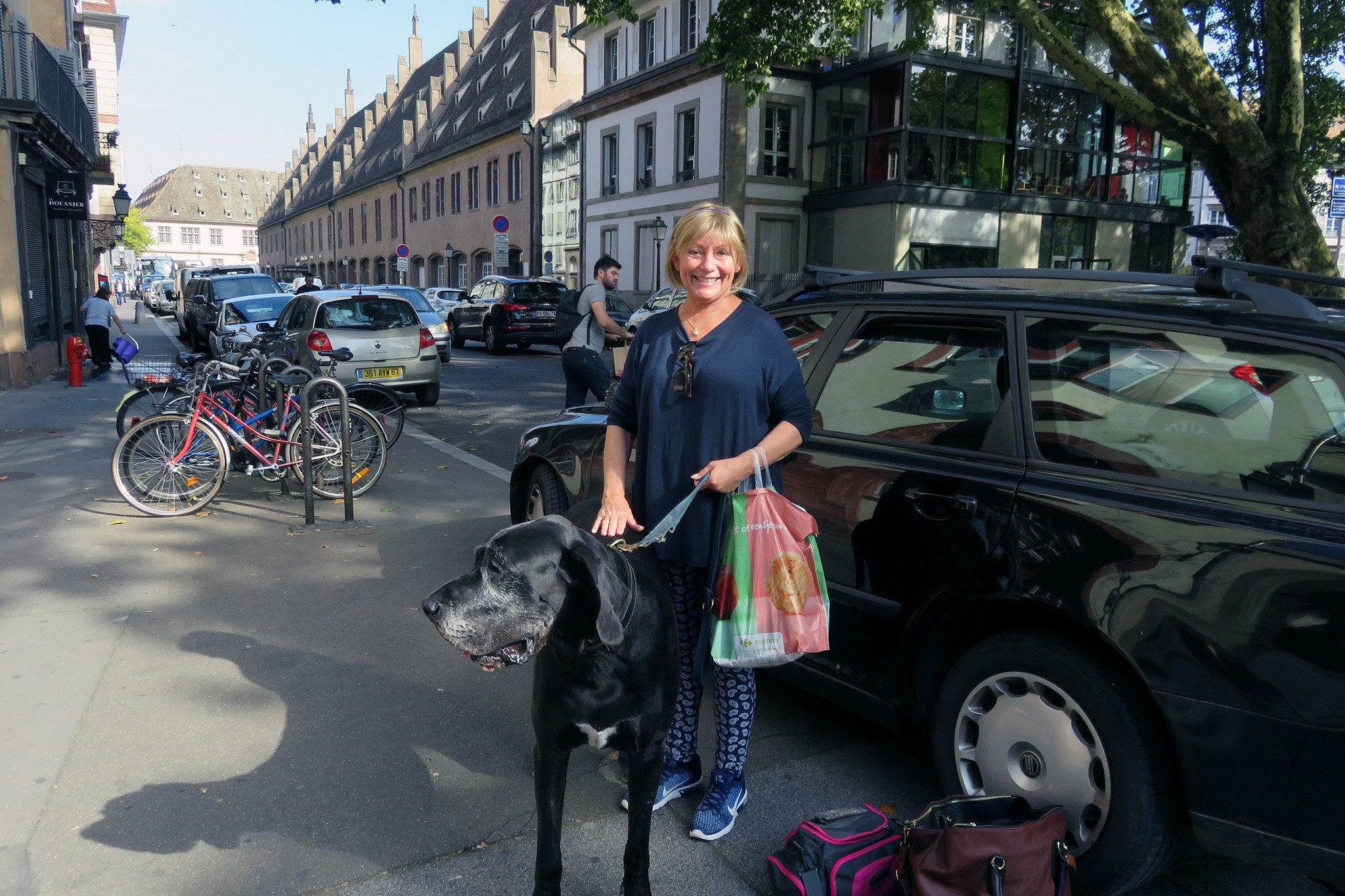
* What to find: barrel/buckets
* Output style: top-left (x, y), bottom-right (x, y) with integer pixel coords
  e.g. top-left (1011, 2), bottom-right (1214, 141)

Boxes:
top-left (111, 332), bottom-right (139, 364)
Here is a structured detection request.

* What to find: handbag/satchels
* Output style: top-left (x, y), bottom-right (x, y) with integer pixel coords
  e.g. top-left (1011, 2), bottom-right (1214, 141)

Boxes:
top-left (711, 446), bottom-right (830, 669)
top-left (896, 795), bottom-right (1077, 896)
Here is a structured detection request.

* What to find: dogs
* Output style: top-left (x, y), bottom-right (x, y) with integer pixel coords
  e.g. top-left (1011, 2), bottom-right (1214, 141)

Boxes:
top-left (417, 495), bottom-right (681, 895)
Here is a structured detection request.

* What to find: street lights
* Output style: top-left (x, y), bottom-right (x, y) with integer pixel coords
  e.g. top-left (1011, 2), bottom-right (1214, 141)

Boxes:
top-left (1182, 223), bottom-right (1239, 259)
top-left (647, 216), bottom-right (668, 291)
top-left (295, 258), bottom-right (300, 275)
top-left (342, 255), bottom-right (349, 284)
top-left (443, 243), bottom-right (455, 290)
top-left (266, 261), bottom-right (271, 274)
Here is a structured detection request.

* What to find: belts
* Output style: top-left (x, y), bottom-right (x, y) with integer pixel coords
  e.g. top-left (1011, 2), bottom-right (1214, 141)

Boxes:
top-left (565, 348), bottom-right (589, 352)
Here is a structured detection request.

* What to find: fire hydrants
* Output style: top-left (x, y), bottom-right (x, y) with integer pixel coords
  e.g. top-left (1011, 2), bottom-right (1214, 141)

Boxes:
top-left (66, 336), bottom-right (90, 387)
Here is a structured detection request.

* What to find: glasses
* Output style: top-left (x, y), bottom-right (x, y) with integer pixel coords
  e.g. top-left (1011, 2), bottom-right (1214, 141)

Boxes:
top-left (673, 340), bottom-right (697, 399)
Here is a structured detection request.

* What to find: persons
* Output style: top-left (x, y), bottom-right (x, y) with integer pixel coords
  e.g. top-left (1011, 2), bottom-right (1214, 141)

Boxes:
top-left (555, 253), bottom-right (635, 410)
top-left (591, 204), bottom-right (811, 841)
top-left (296, 273), bottom-right (321, 294)
top-left (79, 276), bottom-right (146, 372)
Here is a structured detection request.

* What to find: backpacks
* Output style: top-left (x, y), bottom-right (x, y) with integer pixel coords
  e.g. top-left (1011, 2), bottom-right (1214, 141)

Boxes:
top-left (554, 284), bottom-right (596, 352)
top-left (768, 803), bottom-right (904, 896)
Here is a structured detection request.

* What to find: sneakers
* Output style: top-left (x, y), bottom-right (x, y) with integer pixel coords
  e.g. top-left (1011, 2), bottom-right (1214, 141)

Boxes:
top-left (621, 753), bottom-right (703, 812)
top-left (690, 768), bottom-right (748, 841)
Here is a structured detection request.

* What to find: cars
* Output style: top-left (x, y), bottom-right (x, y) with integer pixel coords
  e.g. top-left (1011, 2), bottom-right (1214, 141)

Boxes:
top-left (626, 284), bottom-right (762, 350)
top-left (139, 264), bottom-right (453, 384)
top-left (256, 289), bottom-right (442, 407)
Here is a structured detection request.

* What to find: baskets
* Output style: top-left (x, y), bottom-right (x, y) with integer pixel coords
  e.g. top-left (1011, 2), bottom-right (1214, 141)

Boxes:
top-left (121, 354), bottom-right (180, 387)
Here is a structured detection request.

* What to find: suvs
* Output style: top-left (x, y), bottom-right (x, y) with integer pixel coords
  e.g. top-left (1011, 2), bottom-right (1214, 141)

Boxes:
top-left (447, 274), bottom-right (570, 355)
top-left (509, 254), bottom-right (1344, 896)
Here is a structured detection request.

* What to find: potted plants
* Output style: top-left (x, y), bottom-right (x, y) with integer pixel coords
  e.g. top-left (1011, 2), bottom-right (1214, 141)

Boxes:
top-left (95, 154), bottom-right (110, 172)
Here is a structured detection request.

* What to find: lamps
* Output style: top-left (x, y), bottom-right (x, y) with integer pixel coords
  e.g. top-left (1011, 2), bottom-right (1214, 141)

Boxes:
top-left (79, 183), bottom-right (131, 248)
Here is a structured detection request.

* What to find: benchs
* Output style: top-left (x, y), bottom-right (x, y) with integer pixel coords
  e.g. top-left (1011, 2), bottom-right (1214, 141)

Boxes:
top-left (1016, 176), bottom-right (1061, 194)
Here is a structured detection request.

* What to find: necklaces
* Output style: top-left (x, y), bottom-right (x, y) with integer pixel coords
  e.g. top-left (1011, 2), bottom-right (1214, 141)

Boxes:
top-left (681, 290), bottom-right (733, 337)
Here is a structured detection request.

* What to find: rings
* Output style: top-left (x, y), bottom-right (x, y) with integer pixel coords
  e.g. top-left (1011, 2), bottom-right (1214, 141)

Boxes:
top-left (603, 516), bottom-right (610, 521)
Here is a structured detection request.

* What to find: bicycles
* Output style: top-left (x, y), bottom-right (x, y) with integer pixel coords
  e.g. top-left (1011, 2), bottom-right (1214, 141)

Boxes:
top-left (111, 325), bottom-right (409, 517)
